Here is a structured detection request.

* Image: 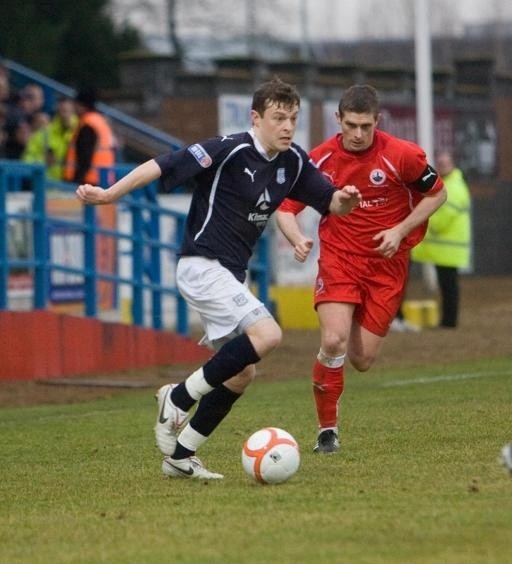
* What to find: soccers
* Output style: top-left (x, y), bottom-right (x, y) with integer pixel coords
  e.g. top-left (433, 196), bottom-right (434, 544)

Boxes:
top-left (243, 427), bottom-right (300, 483)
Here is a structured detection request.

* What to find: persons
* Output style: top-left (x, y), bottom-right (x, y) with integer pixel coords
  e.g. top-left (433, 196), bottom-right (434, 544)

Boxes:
top-left (75, 74), bottom-right (363, 479)
top-left (389, 307), bottom-right (421, 332)
top-left (0, 65), bottom-right (114, 193)
top-left (410, 152), bottom-right (472, 329)
top-left (276, 84), bottom-right (447, 453)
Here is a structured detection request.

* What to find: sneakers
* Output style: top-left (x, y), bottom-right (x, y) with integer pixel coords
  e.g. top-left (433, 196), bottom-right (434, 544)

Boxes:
top-left (155, 383), bottom-right (190, 456)
top-left (313, 426), bottom-right (340, 453)
top-left (161, 455), bottom-right (225, 479)
